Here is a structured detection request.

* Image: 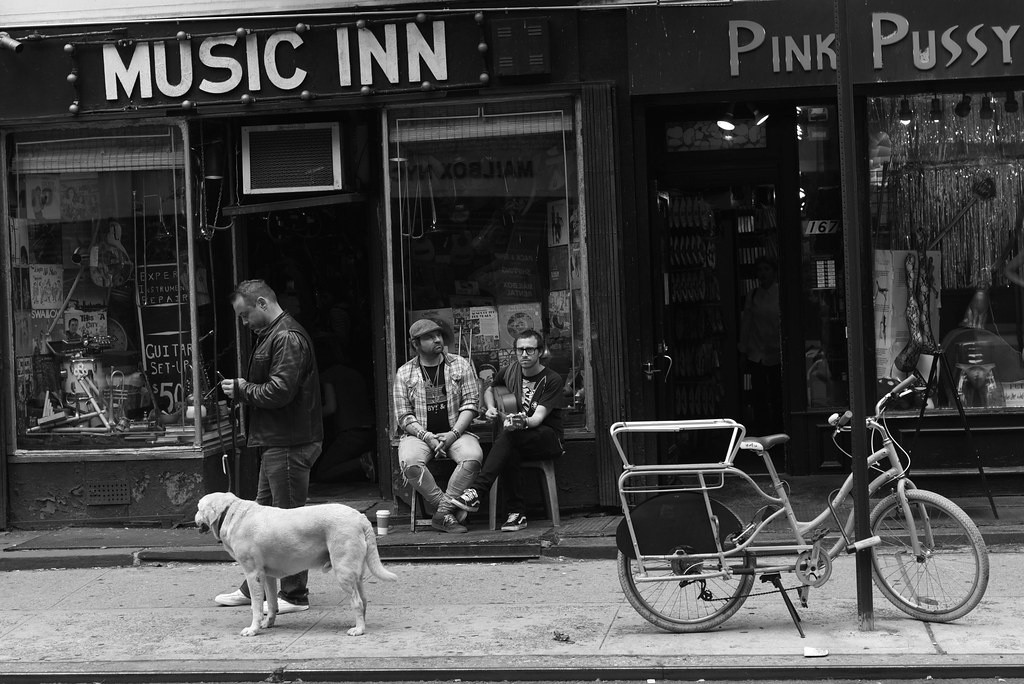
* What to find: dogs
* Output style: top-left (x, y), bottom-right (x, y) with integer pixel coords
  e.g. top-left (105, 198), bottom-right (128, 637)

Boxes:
top-left (194, 491), bottom-right (400, 637)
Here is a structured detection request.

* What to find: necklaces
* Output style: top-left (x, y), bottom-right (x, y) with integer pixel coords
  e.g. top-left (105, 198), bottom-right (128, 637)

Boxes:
top-left (419, 353), bottom-right (441, 404)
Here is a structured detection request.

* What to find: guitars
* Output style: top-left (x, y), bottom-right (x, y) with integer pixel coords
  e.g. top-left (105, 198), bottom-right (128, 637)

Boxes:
top-left (492, 386), bottom-right (523, 429)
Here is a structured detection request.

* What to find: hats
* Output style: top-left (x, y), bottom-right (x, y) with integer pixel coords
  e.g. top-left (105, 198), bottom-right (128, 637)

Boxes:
top-left (409, 319), bottom-right (443, 340)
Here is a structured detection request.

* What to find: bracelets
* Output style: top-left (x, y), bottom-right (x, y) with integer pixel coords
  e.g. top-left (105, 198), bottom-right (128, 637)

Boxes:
top-left (523, 420), bottom-right (529, 430)
top-left (417, 429), bottom-right (432, 442)
top-left (451, 428), bottom-right (460, 439)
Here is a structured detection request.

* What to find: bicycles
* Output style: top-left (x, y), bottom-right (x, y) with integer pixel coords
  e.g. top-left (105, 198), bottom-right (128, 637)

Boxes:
top-left (617, 371), bottom-right (992, 633)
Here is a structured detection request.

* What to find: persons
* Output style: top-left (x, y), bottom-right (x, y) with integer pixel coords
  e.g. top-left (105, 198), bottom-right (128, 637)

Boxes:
top-left (737, 256), bottom-right (784, 460)
top-left (216, 279), bottom-right (325, 615)
top-left (61, 187), bottom-right (97, 220)
top-left (393, 319), bottom-right (483, 534)
top-left (450, 329), bottom-right (564, 531)
top-left (66, 318), bottom-right (82, 342)
top-left (31, 186), bottom-right (44, 219)
top-left (1004, 248), bottom-right (1024, 288)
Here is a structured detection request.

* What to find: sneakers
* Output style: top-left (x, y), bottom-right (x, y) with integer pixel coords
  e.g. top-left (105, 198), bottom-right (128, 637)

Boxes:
top-left (453, 511), bottom-right (467, 523)
top-left (215, 589), bottom-right (251, 605)
top-left (451, 488), bottom-right (480, 512)
top-left (263, 597), bottom-right (309, 614)
top-left (432, 512), bottom-right (467, 533)
top-left (501, 513), bottom-right (527, 531)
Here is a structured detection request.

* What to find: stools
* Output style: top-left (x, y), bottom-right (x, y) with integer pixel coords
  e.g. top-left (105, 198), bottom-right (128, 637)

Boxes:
top-left (408, 457), bottom-right (469, 532)
top-left (488, 459), bottom-right (561, 530)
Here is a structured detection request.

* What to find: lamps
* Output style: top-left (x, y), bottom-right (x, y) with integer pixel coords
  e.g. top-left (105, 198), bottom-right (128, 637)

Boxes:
top-left (980, 93), bottom-right (993, 120)
top-left (929, 94), bottom-right (942, 120)
top-left (898, 95), bottom-right (912, 120)
top-left (716, 100), bottom-right (737, 131)
top-left (746, 101), bottom-right (770, 127)
top-left (955, 94), bottom-right (971, 117)
top-left (205, 145), bottom-right (226, 180)
top-left (389, 145), bottom-right (409, 162)
top-left (1005, 91), bottom-right (1018, 113)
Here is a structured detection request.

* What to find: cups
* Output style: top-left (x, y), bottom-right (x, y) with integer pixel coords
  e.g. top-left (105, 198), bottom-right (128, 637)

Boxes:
top-left (376, 510), bottom-right (390, 534)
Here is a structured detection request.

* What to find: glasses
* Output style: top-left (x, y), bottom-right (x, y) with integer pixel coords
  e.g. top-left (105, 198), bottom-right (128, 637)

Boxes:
top-left (516, 347), bottom-right (538, 355)
